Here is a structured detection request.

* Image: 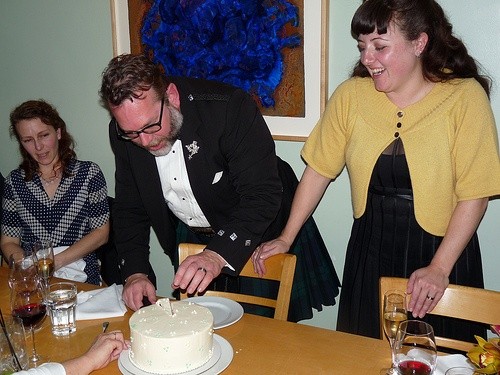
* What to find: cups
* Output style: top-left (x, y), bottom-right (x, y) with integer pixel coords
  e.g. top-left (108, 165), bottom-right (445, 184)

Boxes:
top-left (392, 320), bottom-right (437, 375)
top-left (445, 367), bottom-right (474, 375)
top-left (41, 282), bottom-right (77, 337)
top-left (0, 317), bottom-right (29, 375)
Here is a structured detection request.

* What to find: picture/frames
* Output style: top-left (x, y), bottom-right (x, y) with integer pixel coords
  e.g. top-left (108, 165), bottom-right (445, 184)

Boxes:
top-left (111, 0), bottom-right (330, 142)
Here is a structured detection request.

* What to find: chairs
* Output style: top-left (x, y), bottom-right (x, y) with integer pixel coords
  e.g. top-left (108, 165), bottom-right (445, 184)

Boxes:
top-left (178, 243), bottom-right (297, 321)
top-left (379, 277), bottom-right (500, 360)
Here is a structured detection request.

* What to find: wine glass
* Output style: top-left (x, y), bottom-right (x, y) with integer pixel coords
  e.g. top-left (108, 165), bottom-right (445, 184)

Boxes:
top-left (378, 290), bottom-right (407, 375)
top-left (8, 240), bottom-right (55, 367)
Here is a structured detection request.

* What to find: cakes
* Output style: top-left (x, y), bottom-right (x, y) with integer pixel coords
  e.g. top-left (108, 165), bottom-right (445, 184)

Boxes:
top-left (129, 297), bottom-right (214, 375)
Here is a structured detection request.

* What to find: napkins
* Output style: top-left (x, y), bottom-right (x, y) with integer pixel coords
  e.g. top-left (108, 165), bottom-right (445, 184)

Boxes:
top-left (74, 283), bottom-right (126, 320)
top-left (393, 353), bottom-right (474, 375)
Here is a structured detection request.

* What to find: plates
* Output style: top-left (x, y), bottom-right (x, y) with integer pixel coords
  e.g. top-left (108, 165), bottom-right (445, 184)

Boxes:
top-left (180, 296), bottom-right (244, 330)
top-left (117, 332), bottom-right (234, 375)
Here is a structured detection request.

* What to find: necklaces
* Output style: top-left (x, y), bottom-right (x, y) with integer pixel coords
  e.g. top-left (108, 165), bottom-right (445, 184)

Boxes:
top-left (40, 173), bottom-right (62, 186)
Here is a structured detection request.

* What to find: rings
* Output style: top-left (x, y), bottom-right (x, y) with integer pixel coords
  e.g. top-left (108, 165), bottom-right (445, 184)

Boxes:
top-left (197, 268), bottom-right (206, 272)
top-left (114, 332), bottom-right (116, 339)
top-left (427, 296), bottom-right (434, 300)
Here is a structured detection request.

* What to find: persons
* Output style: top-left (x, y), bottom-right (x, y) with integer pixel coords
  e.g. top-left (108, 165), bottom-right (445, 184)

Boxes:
top-left (250, 0), bottom-right (500, 356)
top-left (9, 329), bottom-right (128, 375)
top-left (97, 51), bottom-right (342, 324)
top-left (0, 101), bottom-right (109, 283)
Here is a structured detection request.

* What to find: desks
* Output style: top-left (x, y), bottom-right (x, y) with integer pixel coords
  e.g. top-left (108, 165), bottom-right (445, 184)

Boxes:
top-left (0, 267), bottom-right (500, 375)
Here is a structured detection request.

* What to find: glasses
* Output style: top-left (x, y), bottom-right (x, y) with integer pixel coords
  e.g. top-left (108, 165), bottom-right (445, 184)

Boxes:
top-left (115, 92), bottom-right (164, 139)
